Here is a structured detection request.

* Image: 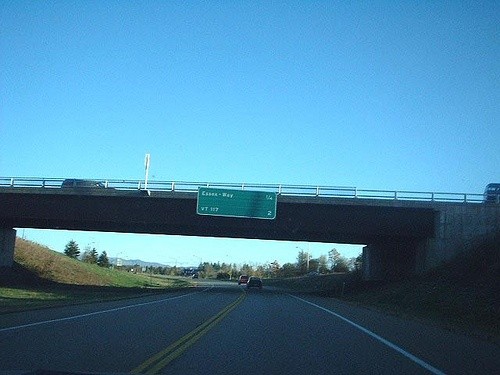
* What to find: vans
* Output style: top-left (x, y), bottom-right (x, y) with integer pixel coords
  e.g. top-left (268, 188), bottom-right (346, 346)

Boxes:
top-left (483, 183), bottom-right (500, 203)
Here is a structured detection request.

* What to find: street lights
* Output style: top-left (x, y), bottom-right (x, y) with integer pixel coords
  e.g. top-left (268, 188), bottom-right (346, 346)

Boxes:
top-left (296, 243), bottom-right (309, 273)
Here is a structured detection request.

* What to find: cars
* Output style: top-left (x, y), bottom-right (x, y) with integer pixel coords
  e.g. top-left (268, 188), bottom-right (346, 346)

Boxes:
top-left (246, 276), bottom-right (262, 288)
top-left (237, 275), bottom-right (249, 285)
top-left (61, 179), bottom-right (104, 187)
